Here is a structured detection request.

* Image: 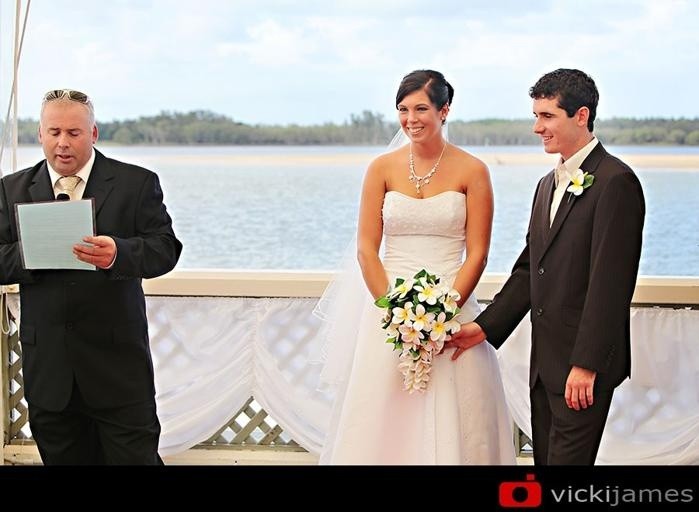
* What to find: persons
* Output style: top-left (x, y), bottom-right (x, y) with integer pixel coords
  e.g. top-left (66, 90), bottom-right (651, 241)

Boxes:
top-left (320, 70), bottom-right (514, 465)
top-left (444, 67), bottom-right (645, 465)
top-left (0, 90), bottom-right (183, 466)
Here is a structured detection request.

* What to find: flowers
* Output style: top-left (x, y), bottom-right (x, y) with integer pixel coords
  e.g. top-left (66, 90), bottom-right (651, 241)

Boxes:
top-left (374, 269), bottom-right (462, 395)
top-left (566, 168), bottom-right (594, 205)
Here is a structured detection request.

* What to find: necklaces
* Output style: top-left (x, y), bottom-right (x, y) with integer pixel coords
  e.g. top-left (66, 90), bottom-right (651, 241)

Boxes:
top-left (408, 141), bottom-right (444, 192)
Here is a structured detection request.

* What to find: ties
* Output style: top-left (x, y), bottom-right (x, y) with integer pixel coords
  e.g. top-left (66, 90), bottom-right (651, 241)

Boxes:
top-left (58, 176), bottom-right (81, 201)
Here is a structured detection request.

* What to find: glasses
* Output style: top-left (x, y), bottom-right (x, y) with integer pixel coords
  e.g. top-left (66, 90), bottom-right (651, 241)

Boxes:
top-left (41, 89), bottom-right (89, 104)
